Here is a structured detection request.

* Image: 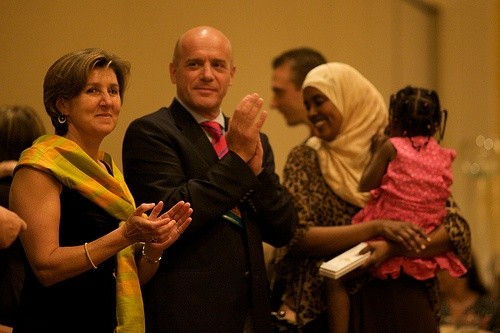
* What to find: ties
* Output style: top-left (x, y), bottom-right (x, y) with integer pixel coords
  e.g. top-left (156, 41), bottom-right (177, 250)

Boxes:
top-left (201, 120), bottom-right (242, 218)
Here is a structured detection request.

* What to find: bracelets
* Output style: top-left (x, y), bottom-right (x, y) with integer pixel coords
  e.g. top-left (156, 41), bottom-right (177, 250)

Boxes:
top-left (84, 242), bottom-right (97, 269)
top-left (142, 244), bottom-right (163, 265)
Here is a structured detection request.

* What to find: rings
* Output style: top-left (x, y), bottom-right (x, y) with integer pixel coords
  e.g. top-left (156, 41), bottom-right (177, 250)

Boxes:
top-left (176, 228), bottom-right (182, 235)
top-left (151, 236), bottom-right (157, 243)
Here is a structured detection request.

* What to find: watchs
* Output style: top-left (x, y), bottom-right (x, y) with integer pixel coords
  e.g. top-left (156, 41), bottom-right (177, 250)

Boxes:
top-left (0, 104), bottom-right (46, 210)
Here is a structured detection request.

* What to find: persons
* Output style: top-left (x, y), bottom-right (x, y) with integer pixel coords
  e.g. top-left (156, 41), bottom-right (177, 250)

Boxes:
top-left (270, 47), bottom-right (327, 137)
top-left (9, 47), bottom-right (193, 333)
top-left (324, 85), bottom-right (457, 333)
top-left (0, 206), bottom-right (27, 333)
top-left (437, 257), bottom-right (500, 333)
top-left (122, 26), bottom-right (299, 333)
top-left (270, 62), bottom-right (472, 333)
